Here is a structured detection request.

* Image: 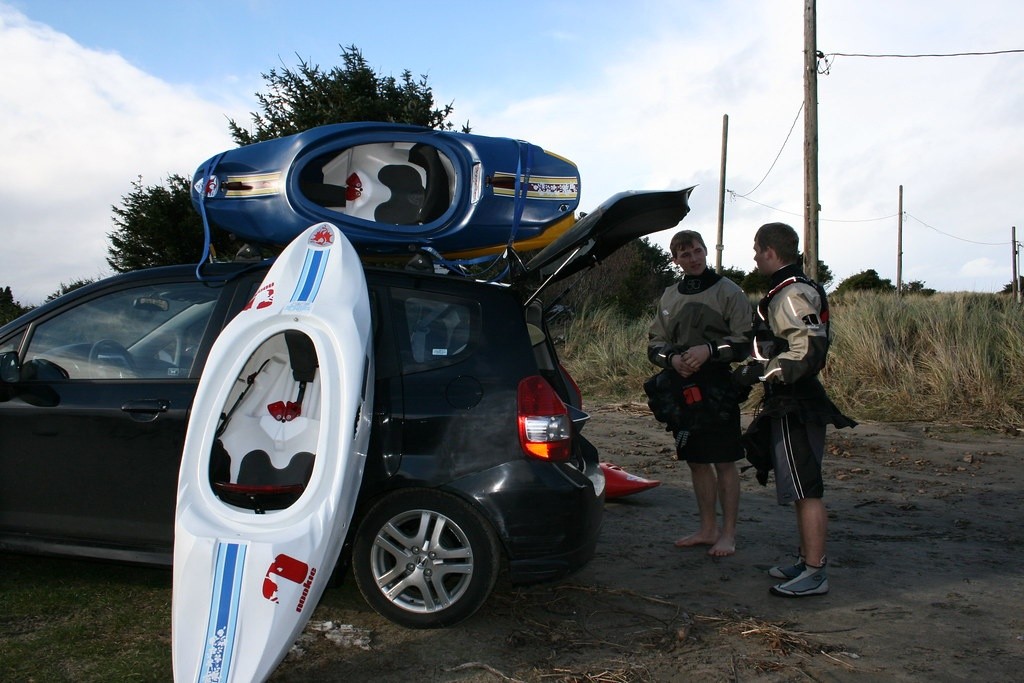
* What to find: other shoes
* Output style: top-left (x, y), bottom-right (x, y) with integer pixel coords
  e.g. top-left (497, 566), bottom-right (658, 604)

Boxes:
top-left (767, 548), bottom-right (807, 580)
top-left (770, 556), bottom-right (829, 598)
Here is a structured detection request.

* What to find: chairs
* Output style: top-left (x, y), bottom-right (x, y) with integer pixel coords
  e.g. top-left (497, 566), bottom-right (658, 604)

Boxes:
top-left (374, 142), bottom-right (450, 226)
top-left (215, 450), bottom-right (315, 509)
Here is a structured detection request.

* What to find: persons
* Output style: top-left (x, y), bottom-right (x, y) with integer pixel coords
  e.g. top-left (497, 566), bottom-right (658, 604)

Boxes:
top-left (739, 223), bottom-right (831, 595)
top-left (647, 230), bottom-right (754, 556)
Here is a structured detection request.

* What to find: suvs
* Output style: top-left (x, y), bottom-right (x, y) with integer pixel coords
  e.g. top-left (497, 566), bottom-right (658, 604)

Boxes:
top-left (0, 184), bottom-right (700, 628)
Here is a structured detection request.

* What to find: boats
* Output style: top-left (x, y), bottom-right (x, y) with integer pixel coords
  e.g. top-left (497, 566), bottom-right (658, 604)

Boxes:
top-left (598, 461), bottom-right (662, 497)
top-left (191, 123), bottom-right (583, 269)
top-left (172, 222), bottom-right (375, 683)
